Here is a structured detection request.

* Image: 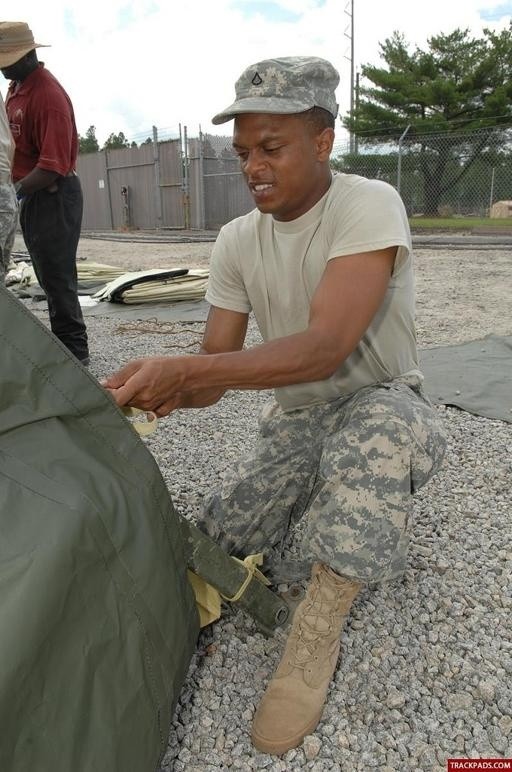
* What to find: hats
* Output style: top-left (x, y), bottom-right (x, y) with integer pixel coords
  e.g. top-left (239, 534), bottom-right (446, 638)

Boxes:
top-left (211, 56), bottom-right (339, 126)
top-left (0, 21), bottom-right (50, 68)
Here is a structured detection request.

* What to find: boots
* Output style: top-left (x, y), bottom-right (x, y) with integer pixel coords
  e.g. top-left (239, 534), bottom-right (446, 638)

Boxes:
top-left (251, 560), bottom-right (365, 755)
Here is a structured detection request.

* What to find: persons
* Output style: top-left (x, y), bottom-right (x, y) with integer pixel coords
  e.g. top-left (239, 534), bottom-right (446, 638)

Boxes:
top-left (1, 23), bottom-right (90, 366)
top-left (1, 97), bottom-right (23, 284)
top-left (102, 57), bottom-right (448, 756)
